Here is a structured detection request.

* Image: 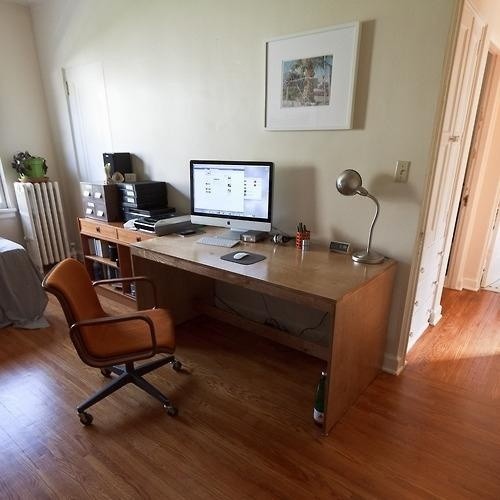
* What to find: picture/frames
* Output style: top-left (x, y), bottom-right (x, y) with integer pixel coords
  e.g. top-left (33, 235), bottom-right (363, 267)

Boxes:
top-left (260, 18), bottom-right (364, 132)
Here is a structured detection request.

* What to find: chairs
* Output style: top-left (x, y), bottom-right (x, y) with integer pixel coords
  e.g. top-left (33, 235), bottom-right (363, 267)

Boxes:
top-left (39, 253), bottom-right (184, 428)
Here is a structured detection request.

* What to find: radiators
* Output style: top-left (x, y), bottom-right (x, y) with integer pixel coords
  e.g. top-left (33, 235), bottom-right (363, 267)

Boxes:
top-left (12, 180), bottom-right (72, 269)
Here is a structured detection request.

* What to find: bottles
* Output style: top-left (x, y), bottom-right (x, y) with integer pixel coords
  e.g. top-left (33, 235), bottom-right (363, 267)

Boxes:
top-left (108, 245), bottom-right (117, 261)
top-left (30, 159), bottom-right (43, 179)
top-left (312, 370), bottom-right (326, 429)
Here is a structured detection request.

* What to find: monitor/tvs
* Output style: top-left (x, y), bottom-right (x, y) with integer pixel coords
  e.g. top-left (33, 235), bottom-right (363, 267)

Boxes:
top-left (190, 160), bottom-right (273, 239)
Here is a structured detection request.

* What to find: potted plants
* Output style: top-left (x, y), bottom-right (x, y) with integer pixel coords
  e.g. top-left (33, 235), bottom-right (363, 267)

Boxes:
top-left (8, 150), bottom-right (49, 184)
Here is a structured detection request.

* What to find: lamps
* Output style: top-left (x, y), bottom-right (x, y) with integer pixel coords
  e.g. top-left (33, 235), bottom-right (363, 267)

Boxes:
top-left (334, 166), bottom-right (388, 265)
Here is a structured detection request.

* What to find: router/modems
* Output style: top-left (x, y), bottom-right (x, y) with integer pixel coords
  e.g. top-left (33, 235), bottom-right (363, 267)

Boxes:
top-left (240, 229), bottom-right (268, 242)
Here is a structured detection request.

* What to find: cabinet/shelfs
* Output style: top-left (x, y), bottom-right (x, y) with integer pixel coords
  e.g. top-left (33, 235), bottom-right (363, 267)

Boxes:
top-left (76, 217), bottom-right (160, 311)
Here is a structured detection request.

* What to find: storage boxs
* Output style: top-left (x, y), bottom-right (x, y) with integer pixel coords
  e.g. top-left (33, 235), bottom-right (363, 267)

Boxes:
top-left (80, 181), bottom-right (124, 223)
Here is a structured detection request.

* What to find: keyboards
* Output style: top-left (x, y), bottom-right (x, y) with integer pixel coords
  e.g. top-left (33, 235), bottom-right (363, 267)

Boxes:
top-left (196, 237), bottom-right (241, 248)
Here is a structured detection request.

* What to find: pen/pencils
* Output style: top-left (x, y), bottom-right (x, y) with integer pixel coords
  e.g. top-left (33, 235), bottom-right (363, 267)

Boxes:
top-left (296, 222), bottom-right (306, 232)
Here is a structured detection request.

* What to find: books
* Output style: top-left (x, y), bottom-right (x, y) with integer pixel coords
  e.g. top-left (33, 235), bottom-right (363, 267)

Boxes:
top-left (80, 237), bottom-right (136, 298)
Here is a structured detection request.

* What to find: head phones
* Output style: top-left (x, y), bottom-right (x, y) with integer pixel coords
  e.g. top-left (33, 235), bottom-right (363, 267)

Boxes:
top-left (271, 234), bottom-right (296, 244)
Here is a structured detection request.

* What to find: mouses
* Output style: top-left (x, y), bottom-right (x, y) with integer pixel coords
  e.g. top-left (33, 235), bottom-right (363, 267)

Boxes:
top-left (233, 252), bottom-right (250, 260)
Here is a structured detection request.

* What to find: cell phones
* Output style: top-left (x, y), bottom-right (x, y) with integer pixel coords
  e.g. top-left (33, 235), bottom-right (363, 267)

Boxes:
top-left (180, 230), bottom-right (196, 235)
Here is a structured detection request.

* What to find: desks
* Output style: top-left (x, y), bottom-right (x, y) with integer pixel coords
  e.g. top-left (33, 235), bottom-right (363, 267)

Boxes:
top-left (0, 237), bottom-right (49, 328)
top-left (131, 220), bottom-right (396, 433)
